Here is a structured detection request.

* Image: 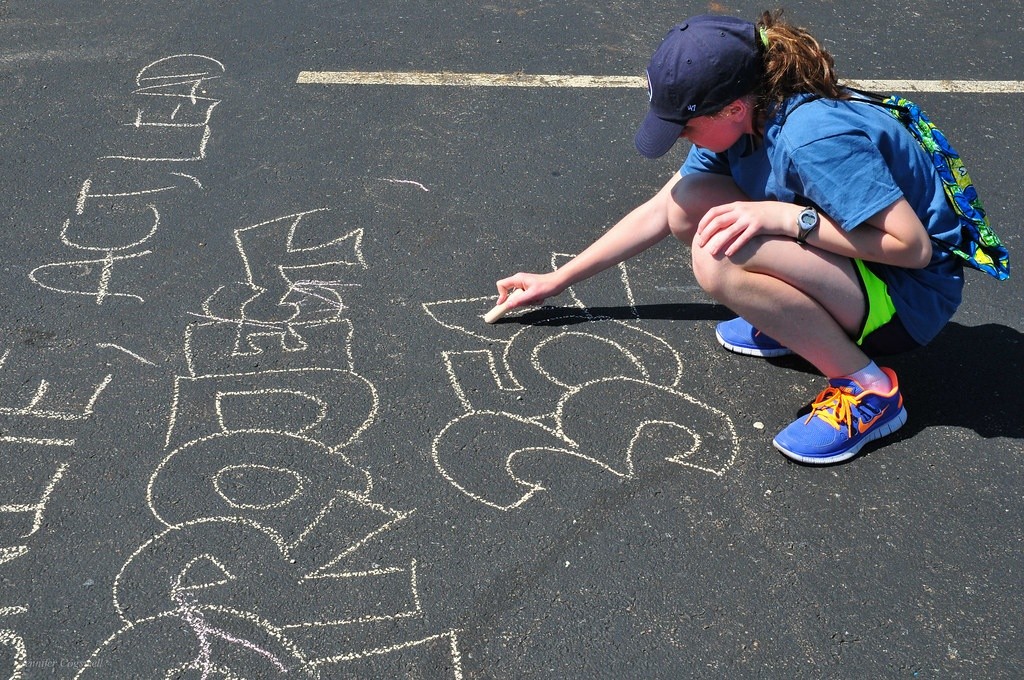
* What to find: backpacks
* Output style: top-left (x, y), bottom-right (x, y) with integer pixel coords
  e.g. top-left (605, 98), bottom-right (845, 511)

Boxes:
top-left (779, 83), bottom-right (1012, 281)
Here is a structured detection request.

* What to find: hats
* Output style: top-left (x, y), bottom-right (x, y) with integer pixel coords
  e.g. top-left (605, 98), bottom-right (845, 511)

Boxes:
top-left (633, 14), bottom-right (756, 160)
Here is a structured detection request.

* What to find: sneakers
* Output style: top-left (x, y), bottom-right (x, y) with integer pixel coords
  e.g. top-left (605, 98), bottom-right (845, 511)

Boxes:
top-left (715, 316), bottom-right (797, 357)
top-left (773, 366), bottom-right (908, 464)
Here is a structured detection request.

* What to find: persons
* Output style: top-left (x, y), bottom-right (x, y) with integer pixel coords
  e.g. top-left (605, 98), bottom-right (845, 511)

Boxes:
top-left (496, 14), bottom-right (1010, 464)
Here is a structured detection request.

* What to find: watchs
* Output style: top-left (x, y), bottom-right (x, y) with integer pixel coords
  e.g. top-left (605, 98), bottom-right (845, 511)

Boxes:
top-left (797, 206), bottom-right (819, 247)
